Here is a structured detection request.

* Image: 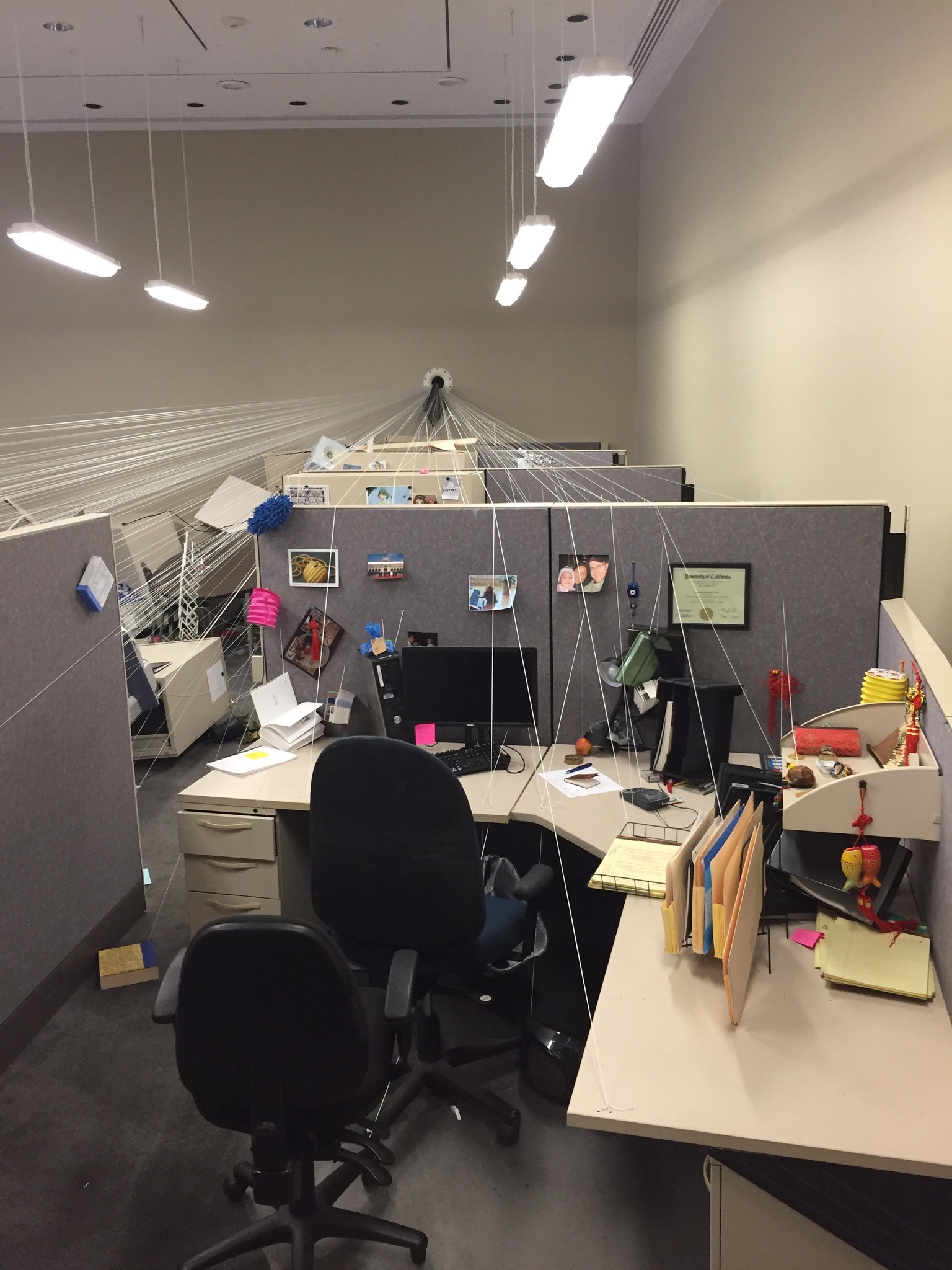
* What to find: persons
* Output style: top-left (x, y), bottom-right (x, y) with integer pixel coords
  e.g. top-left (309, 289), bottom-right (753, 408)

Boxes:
top-left (377, 569), bottom-right (398, 577)
top-left (581, 555), bottom-right (609, 593)
top-left (426, 638), bottom-right (436, 647)
top-left (557, 566), bottom-right (577, 592)
top-left (477, 585), bottom-right (496, 610)
top-left (408, 633), bottom-right (415, 647)
top-left (571, 561), bottom-right (591, 591)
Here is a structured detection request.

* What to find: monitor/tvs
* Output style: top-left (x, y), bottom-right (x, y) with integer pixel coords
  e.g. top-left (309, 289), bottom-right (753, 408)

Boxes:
top-left (401, 646), bottom-right (538, 728)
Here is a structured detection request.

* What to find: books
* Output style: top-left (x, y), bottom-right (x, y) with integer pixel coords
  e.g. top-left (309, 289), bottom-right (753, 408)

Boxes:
top-left (249, 671), bottom-right (326, 754)
top-left (97, 939), bottom-right (160, 992)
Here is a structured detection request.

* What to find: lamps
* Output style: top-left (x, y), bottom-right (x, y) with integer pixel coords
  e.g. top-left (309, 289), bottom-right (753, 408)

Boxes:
top-left (0, 0), bottom-right (123, 278)
top-left (488, 0), bottom-right (633, 309)
top-left (132, 3), bottom-right (210, 310)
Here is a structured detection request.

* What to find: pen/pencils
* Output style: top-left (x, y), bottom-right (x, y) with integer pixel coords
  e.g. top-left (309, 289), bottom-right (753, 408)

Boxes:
top-left (674, 779), bottom-right (689, 786)
top-left (701, 781), bottom-right (713, 789)
top-left (703, 787), bottom-right (716, 796)
top-left (564, 763), bottom-right (592, 774)
top-left (666, 779), bottom-right (672, 794)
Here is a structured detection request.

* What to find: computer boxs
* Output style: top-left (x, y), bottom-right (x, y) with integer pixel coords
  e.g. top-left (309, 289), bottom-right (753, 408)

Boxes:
top-left (357, 647), bottom-right (418, 746)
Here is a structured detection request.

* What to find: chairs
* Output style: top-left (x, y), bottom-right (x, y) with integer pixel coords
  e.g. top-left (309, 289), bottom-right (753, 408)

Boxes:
top-left (305, 735), bottom-right (559, 1140)
top-left (159, 916), bottom-right (429, 1270)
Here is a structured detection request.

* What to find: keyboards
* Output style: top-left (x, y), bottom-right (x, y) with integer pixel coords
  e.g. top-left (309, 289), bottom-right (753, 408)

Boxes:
top-left (430, 742), bottom-right (505, 777)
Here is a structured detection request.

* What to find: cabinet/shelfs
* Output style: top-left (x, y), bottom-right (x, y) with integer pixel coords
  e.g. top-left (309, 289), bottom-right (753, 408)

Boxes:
top-left (174, 743), bottom-right (307, 919)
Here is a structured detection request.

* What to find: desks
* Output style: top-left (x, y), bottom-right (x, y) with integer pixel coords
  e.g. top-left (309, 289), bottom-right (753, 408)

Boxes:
top-left (563, 889), bottom-right (952, 1270)
